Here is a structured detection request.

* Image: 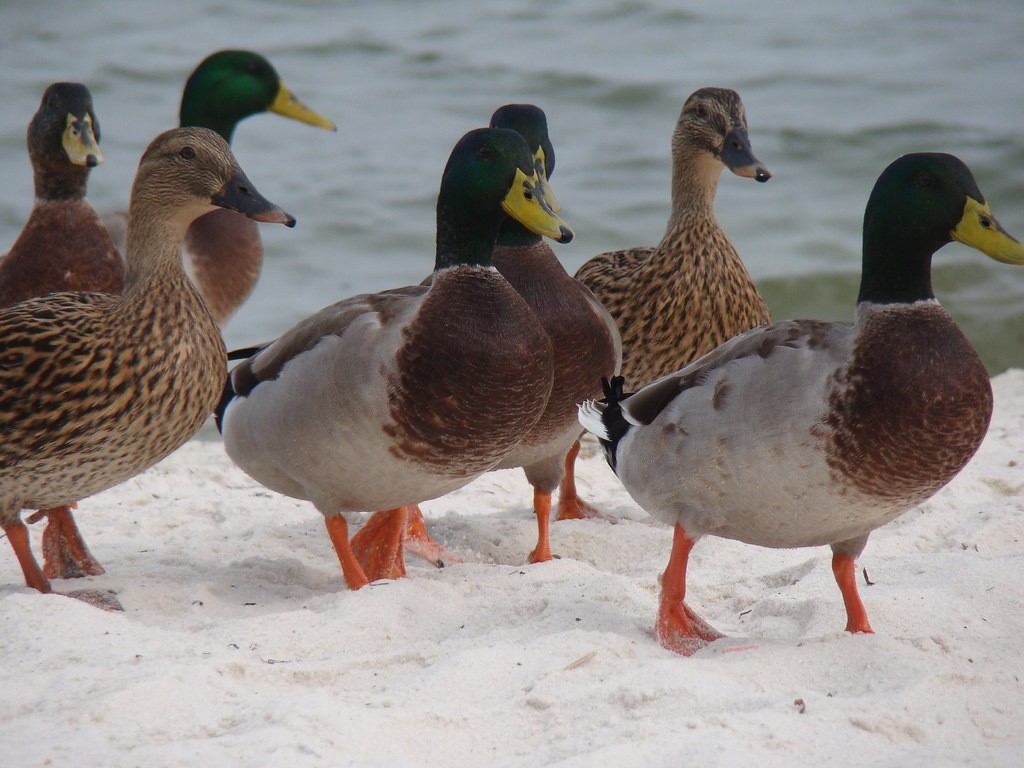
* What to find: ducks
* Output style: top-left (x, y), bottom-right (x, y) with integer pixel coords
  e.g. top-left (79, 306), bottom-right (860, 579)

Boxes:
top-left (0, 50), bottom-right (1024, 656)
